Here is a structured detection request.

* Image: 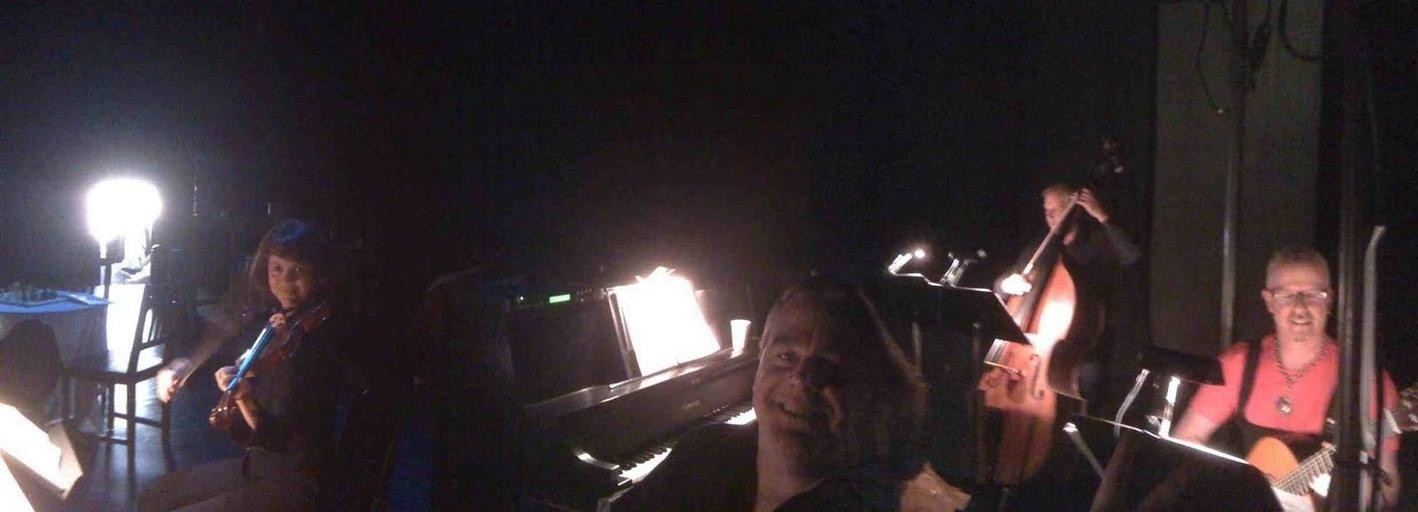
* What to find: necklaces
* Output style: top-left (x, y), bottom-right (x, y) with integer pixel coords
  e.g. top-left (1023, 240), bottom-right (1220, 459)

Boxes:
top-left (1267, 333), bottom-right (1329, 416)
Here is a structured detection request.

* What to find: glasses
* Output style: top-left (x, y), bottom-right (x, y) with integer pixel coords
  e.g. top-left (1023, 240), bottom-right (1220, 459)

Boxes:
top-left (1267, 284), bottom-right (1332, 304)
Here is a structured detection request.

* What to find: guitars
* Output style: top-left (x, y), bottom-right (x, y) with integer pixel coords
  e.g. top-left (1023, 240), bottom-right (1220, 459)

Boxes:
top-left (1246, 381), bottom-right (1418, 512)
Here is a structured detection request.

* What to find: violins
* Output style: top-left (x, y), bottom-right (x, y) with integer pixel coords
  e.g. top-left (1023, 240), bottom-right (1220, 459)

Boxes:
top-left (209, 294), bottom-right (334, 432)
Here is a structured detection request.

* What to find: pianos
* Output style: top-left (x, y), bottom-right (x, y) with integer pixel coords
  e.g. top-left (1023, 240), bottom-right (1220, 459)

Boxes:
top-left (488, 271), bottom-right (758, 510)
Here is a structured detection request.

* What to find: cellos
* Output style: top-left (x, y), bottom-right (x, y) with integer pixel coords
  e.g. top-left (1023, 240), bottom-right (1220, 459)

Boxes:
top-left (945, 137), bottom-right (1128, 484)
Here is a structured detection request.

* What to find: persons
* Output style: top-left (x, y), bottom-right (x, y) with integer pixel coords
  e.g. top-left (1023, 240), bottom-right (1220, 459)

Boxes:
top-left (988, 180), bottom-right (1146, 417)
top-left (1165, 239), bottom-right (1403, 512)
top-left (608, 261), bottom-right (871, 510)
top-left (136, 213), bottom-right (344, 511)
